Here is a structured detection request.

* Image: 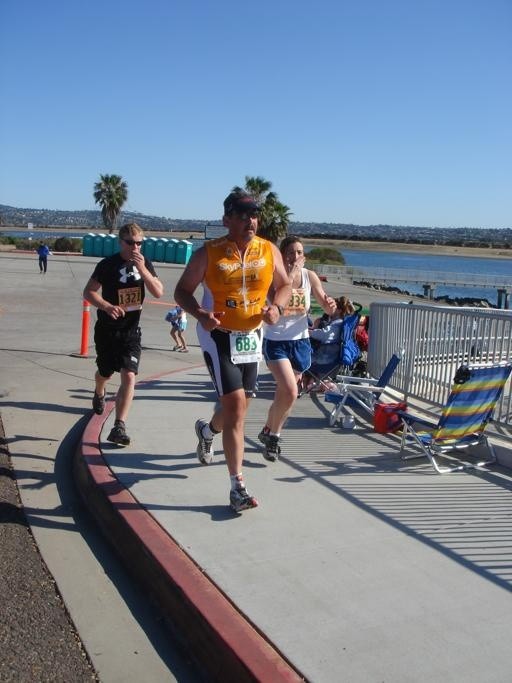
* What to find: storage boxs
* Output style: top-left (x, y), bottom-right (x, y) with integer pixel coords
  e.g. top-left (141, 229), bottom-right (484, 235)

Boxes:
top-left (371, 398), bottom-right (409, 433)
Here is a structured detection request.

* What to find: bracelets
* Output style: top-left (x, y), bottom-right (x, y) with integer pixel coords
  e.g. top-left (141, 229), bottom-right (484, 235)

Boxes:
top-left (274, 302), bottom-right (285, 317)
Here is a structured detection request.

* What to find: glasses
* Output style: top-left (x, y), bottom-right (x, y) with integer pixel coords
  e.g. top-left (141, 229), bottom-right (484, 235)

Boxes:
top-left (233, 211), bottom-right (259, 219)
top-left (122, 239), bottom-right (142, 246)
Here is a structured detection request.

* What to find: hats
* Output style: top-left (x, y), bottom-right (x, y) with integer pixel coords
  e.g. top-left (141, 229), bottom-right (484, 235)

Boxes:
top-left (223, 191), bottom-right (262, 214)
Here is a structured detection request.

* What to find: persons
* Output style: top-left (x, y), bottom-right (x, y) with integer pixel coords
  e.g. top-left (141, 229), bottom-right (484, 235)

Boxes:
top-left (166, 304), bottom-right (191, 352)
top-left (296, 294), bottom-right (354, 401)
top-left (35, 239), bottom-right (53, 275)
top-left (82, 222), bottom-right (165, 445)
top-left (175, 185), bottom-right (294, 512)
top-left (258, 234), bottom-right (337, 462)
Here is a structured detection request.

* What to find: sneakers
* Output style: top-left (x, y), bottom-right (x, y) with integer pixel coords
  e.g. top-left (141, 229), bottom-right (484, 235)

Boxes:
top-left (263, 430), bottom-right (279, 461)
top-left (92, 388), bottom-right (106, 415)
top-left (258, 430), bottom-right (282, 454)
top-left (107, 423), bottom-right (130, 444)
top-left (195, 418), bottom-right (215, 465)
top-left (229, 487), bottom-right (258, 513)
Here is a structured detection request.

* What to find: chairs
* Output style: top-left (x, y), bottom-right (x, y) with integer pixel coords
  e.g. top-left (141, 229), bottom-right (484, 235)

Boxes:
top-left (322, 346), bottom-right (407, 429)
top-left (296, 312), bottom-right (361, 400)
top-left (396, 357), bottom-right (511, 476)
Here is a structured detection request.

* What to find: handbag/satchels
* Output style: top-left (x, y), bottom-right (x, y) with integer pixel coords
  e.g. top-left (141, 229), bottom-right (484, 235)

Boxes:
top-left (372, 402), bottom-right (408, 433)
